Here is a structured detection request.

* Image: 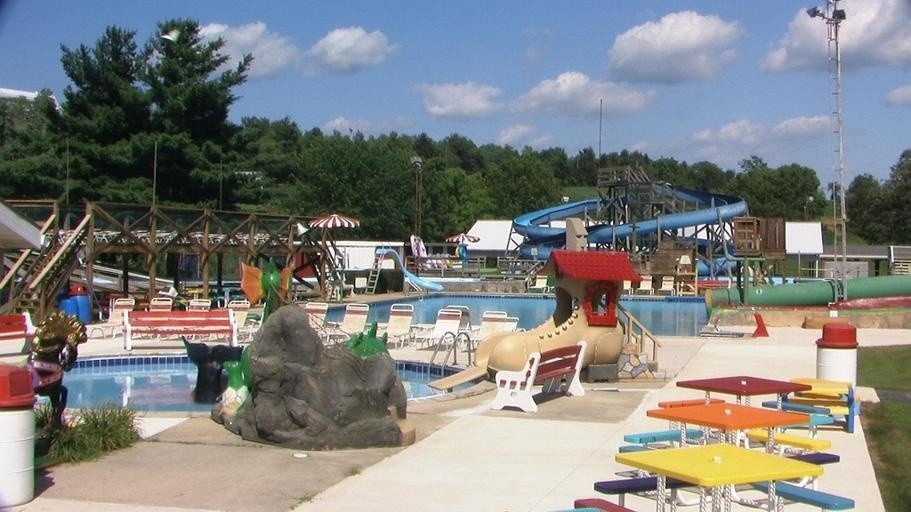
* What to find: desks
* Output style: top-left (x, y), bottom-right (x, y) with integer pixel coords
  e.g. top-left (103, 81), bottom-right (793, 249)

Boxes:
top-left (646, 402), bottom-right (811, 455)
top-left (676, 375), bottom-right (812, 412)
top-left (614, 442), bottom-right (825, 512)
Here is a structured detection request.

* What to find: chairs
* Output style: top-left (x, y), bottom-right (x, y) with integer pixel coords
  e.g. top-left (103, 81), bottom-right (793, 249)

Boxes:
top-left (90, 296), bottom-right (523, 354)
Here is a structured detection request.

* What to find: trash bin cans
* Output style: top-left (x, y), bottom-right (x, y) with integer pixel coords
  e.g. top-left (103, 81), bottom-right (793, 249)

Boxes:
top-left (0, 362), bottom-right (37, 508)
top-left (815, 322), bottom-right (859, 419)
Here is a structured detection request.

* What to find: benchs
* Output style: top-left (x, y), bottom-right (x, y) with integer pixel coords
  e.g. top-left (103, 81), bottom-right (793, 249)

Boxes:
top-left (0, 308), bottom-right (45, 358)
top-left (491, 336), bottom-right (590, 419)
top-left (575, 374), bottom-right (867, 512)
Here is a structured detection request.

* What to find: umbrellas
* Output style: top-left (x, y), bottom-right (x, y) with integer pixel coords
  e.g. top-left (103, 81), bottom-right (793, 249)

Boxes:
top-left (309, 214), bottom-right (361, 254)
top-left (446, 233), bottom-right (481, 257)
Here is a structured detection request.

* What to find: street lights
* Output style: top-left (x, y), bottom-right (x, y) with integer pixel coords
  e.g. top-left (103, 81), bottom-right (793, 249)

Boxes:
top-left (409, 154), bottom-right (422, 276)
top-left (807, 0), bottom-right (853, 302)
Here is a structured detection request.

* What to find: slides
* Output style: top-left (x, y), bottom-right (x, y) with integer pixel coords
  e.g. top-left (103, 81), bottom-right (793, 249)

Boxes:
top-left (513, 182), bottom-right (748, 277)
